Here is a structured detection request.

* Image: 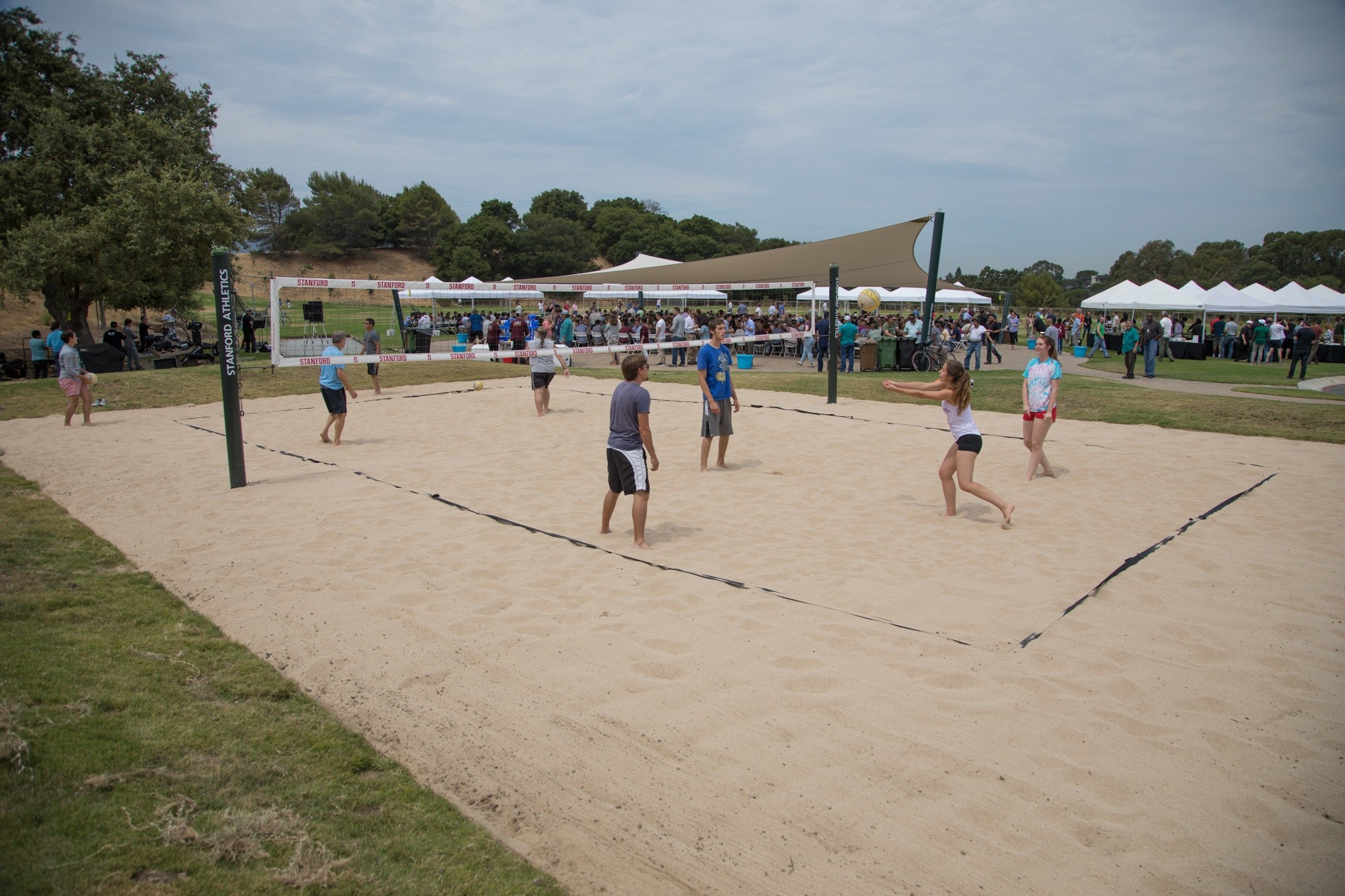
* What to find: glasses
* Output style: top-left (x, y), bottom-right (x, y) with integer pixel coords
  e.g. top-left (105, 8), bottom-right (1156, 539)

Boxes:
top-left (246, 309), bottom-right (252, 312)
top-left (690, 313), bottom-right (695, 315)
top-left (1147, 315), bottom-right (1151, 317)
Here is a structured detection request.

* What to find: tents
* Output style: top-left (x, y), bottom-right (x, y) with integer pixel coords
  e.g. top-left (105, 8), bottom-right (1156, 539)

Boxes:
top-left (795, 281), bottom-right (991, 319)
top-left (1080, 279), bottom-right (1345, 343)
top-left (393, 276), bottom-right (544, 321)
top-left (582, 290), bottom-right (728, 312)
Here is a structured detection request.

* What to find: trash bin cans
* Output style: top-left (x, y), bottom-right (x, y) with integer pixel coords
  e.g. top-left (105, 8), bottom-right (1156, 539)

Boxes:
top-left (928, 344), bottom-right (948, 371)
top-left (897, 336), bottom-right (918, 371)
top-left (859, 340), bottom-right (878, 372)
top-left (874, 336), bottom-right (897, 372)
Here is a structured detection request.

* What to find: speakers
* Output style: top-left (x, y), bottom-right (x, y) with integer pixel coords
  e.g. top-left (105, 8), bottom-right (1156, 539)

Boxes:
top-left (303, 301), bottom-right (323, 322)
top-left (258, 344), bottom-right (271, 353)
top-left (193, 330), bottom-right (203, 354)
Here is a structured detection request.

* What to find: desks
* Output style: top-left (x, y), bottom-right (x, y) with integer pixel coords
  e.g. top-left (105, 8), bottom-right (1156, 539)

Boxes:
top-left (436, 321), bottom-right (457, 324)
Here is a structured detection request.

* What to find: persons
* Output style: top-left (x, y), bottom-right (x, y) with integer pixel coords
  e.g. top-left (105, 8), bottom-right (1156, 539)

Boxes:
top-left (139, 316), bottom-right (157, 356)
top-left (523, 325), bottom-right (570, 418)
top-left (102, 318), bottom-right (144, 371)
top-left (160, 308), bottom-right (177, 339)
top-left (319, 330), bottom-right (357, 446)
top-left (1022, 335), bottom-right (1062, 482)
top-left (29, 321), bottom-right (65, 379)
top-left (404, 298), bottom-right (1345, 379)
top-left (882, 360), bottom-right (1014, 525)
top-left (58, 330), bottom-right (94, 427)
top-left (698, 318), bottom-right (740, 473)
top-left (360, 318), bottom-right (382, 395)
top-left (601, 354), bottom-right (660, 550)
top-left (240, 309), bottom-right (259, 353)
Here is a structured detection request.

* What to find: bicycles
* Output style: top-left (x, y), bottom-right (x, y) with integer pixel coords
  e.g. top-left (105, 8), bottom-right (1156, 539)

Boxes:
top-left (911, 341), bottom-right (957, 373)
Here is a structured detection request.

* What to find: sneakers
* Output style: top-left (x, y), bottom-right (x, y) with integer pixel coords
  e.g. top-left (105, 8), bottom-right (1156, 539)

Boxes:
top-left (92, 398), bottom-right (107, 406)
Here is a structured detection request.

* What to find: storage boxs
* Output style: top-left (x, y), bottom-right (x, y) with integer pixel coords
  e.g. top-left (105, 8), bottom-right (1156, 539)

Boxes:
top-left (553, 344), bottom-right (570, 367)
top-left (471, 344), bottom-right (501, 361)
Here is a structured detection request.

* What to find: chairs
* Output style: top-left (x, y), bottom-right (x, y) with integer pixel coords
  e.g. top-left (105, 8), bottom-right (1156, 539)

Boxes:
top-left (572, 331), bottom-right (799, 358)
top-left (423, 320), bottom-right (458, 335)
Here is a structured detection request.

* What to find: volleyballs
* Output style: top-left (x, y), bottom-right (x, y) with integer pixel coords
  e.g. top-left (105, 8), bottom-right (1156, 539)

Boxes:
top-left (857, 288), bottom-right (881, 312)
top-left (84, 373), bottom-right (97, 385)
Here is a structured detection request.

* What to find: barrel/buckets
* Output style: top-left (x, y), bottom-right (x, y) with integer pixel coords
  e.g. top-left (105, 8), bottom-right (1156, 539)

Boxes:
top-left (736, 354), bottom-right (754, 369)
top-left (456, 333), bottom-right (468, 343)
top-left (1027, 339), bottom-right (1037, 349)
top-left (1073, 346), bottom-right (1088, 357)
top-left (452, 343), bottom-right (466, 352)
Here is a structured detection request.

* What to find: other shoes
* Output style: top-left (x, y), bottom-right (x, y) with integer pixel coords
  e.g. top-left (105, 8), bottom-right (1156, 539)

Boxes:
top-left (1247, 360), bottom-right (1250, 363)
top-left (657, 362), bottom-right (666, 366)
top-left (1234, 359), bottom-right (1239, 362)
top-left (668, 364), bottom-right (678, 367)
top-left (1011, 345), bottom-right (1015, 350)
top-left (998, 356), bottom-right (1002, 363)
top-left (1170, 359), bottom-right (1175, 362)
top-left (678, 364), bottom-right (684, 367)
top-left (1143, 375), bottom-right (1155, 379)
top-left (795, 361), bottom-right (802, 366)
top-left (687, 361), bottom-right (697, 364)
top-left (808, 365), bottom-right (814, 367)
top-left (1286, 375), bottom-right (1293, 379)
top-left (1299, 377), bottom-right (1304, 381)
top-left (1279, 362), bottom-right (1285, 365)
top-left (610, 361), bottom-right (620, 365)
top-left (984, 362), bottom-right (991, 364)
top-left (1122, 375), bottom-right (1135, 378)
top-left (1157, 357), bottom-right (1162, 362)
top-left (1250, 363), bottom-right (1254, 365)
top-left (1065, 345), bottom-right (1068, 348)
top-left (1217, 356), bottom-right (1224, 359)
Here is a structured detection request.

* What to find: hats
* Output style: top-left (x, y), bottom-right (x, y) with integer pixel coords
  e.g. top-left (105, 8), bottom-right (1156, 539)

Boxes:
top-left (1039, 307), bottom-right (1043, 310)
top-left (1258, 319), bottom-right (1265, 324)
top-left (1246, 320), bottom-right (1253, 324)
top-left (562, 309), bottom-right (569, 314)
top-left (1267, 318), bottom-right (1273, 321)
top-left (471, 308), bottom-right (479, 314)
top-left (844, 315), bottom-right (852, 321)
